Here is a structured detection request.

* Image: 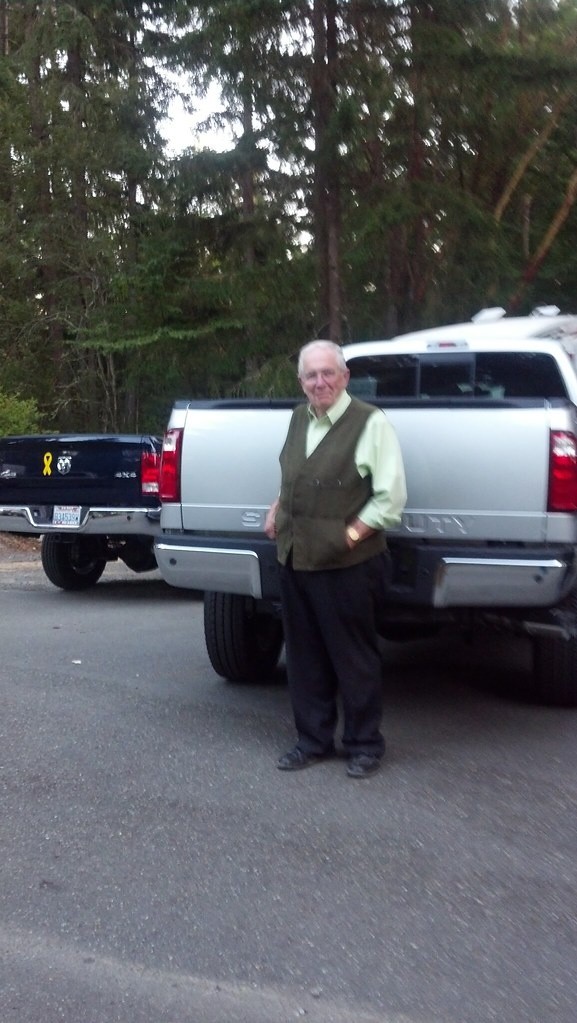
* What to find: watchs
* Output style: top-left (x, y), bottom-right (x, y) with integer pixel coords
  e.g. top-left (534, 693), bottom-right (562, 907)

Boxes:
top-left (347, 525), bottom-right (362, 545)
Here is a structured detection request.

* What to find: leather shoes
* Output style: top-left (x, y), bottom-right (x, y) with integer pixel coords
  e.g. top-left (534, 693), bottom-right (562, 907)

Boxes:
top-left (277, 743), bottom-right (329, 770)
top-left (346, 753), bottom-right (381, 776)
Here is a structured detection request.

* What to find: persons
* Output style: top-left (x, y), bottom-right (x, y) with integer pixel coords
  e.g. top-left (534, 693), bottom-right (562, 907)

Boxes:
top-left (264, 341), bottom-right (408, 778)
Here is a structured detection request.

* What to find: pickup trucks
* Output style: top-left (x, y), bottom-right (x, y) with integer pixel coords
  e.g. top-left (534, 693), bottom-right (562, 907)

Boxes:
top-left (0, 433), bottom-right (164, 591)
top-left (154, 306), bottom-right (576, 709)
